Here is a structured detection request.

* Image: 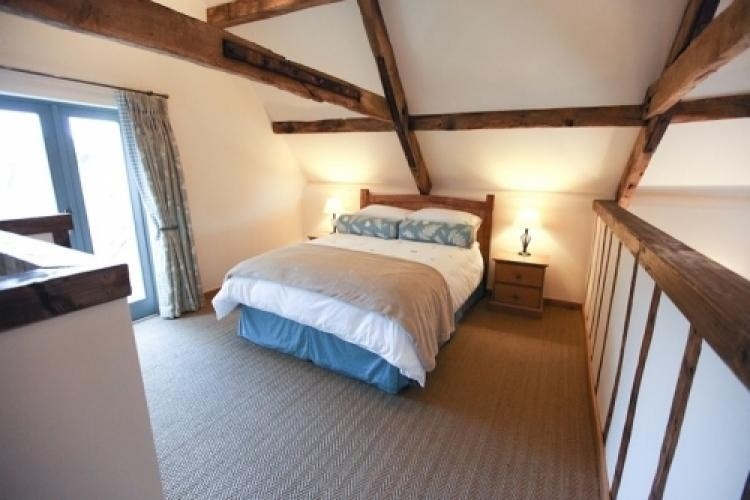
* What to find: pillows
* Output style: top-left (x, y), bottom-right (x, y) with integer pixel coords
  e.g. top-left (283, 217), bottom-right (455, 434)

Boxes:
top-left (333, 214), bottom-right (403, 240)
top-left (398, 220), bottom-right (475, 249)
top-left (406, 207), bottom-right (484, 229)
top-left (352, 203), bottom-right (416, 222)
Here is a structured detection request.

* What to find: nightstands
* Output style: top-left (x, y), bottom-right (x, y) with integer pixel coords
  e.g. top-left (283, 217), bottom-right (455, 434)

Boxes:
top-left (491, 252), bottom-right (550, 320)
top-left (307, 236), bottom-right (317, 240)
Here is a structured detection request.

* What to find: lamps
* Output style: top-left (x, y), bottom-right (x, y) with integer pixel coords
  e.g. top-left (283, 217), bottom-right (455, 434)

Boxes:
top-left (520, 229), bottom-right (531, 257)
top-left (324, 192), bottom-right (344, 235)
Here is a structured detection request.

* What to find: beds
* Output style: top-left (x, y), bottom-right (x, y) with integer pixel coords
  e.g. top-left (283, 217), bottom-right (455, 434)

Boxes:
top-left (212, 188), bottom-right (495, 396)
